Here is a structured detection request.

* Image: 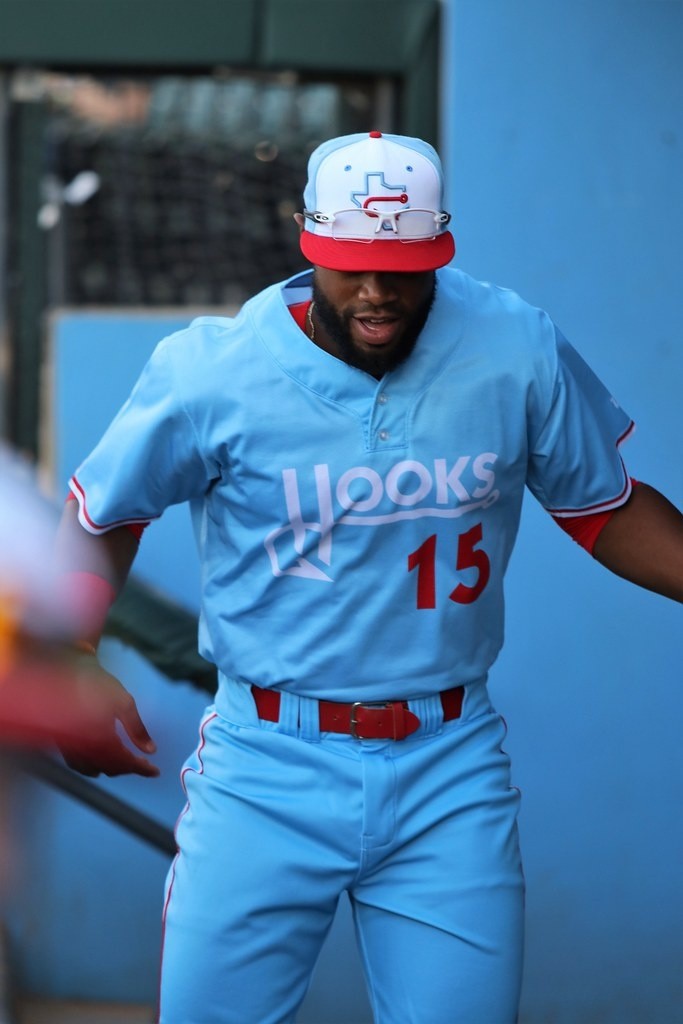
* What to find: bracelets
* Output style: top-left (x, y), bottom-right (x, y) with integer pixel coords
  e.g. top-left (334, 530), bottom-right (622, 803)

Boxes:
top-left (69, 639), bottom-right (96, 654)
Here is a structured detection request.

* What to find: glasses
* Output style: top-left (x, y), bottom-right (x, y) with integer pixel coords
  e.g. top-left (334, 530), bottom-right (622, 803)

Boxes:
top-left (303, 206), bottom-right (451, 244)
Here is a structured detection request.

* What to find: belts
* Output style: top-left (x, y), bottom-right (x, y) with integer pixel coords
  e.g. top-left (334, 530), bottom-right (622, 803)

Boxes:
top-left (254, 686), bottom-right (467, 741)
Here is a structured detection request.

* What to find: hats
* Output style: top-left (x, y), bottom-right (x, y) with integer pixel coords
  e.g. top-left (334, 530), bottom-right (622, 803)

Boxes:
top-left (301, 130), bottom-right (458, 272)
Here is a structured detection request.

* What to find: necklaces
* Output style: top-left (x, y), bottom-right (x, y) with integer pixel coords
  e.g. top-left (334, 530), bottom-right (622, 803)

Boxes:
top-left (307, 301), bottom-right (315, 340)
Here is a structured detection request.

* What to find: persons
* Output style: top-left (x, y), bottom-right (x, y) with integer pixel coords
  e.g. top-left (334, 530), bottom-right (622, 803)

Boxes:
top-left (49, 130), bottom-right (683, 1023)
